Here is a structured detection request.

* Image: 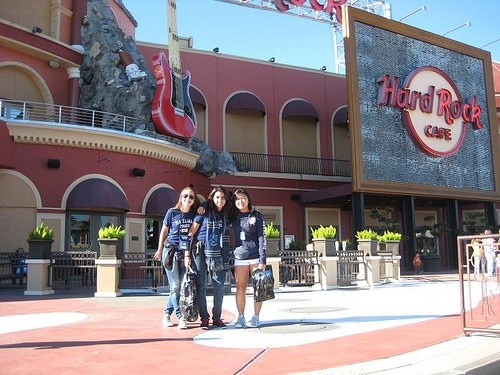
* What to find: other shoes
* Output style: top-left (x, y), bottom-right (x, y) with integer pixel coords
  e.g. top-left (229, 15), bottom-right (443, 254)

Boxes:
top-left (162, 316), bottom-right (173, 327)
top-left (179, 317), bottom-right (187, 328)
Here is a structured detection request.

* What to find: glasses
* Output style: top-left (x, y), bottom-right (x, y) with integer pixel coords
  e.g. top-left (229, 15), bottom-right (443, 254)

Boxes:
top-left (182, 194), bottom-right (195, 200)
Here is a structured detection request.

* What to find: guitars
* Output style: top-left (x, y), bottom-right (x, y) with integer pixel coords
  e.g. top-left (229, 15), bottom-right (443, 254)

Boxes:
top-left (151, 1), bottom-right (197, 143)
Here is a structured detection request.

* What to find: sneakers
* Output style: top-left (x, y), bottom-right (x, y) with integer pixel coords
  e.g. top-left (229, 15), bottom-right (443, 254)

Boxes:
top-left (200, 318), bottom-right (210, 329)
top-left (213, 319), bottom-right (227, 329)
top-left (247, 316), bottom-right (260, 328)
top-left (235, 316), bottom-right (245, 328)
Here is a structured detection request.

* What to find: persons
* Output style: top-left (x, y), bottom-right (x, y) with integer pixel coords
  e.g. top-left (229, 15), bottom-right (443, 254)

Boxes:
top-left (197, 188), bottom-right (266, 328)
top-left (413, 252), bottom-right (422, 276)
top-left (183, 186), bottom-right (228, 329)
top-left (471, 229), bottom-right (496, 280)
top-left (153, 186), bottom-right (195, 330)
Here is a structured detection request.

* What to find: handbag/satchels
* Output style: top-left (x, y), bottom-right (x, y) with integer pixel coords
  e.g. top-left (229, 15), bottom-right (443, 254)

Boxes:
top-left (251, 264), bottom-right (275, 303)
top-left (179, 265), bottom-right (199, 322)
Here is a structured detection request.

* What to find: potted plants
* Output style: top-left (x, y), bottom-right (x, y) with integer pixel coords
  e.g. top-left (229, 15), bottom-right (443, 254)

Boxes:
top-left (97, 224), bottom-right (126, 259)
top-left (265, 222), bottom-right (280, 256)
top-left (27, 222), bottom-right (54, 258)
top-left (355, 228), bottom-right (378, 256)
top-left (310, 225), bottom-right (337, 256)
top-left (383, 230), bottom-right (401, 254)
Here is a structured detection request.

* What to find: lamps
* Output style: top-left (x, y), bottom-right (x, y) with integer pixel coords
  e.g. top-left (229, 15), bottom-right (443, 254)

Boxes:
top-left (321, 66), bottom-right (327, 71)
top-left (270, 57), bottom-right (275, 63)
top-left (213, 47), bottom-right (219, 52)
top-left (32, 26), bottom-right (42, 34)
